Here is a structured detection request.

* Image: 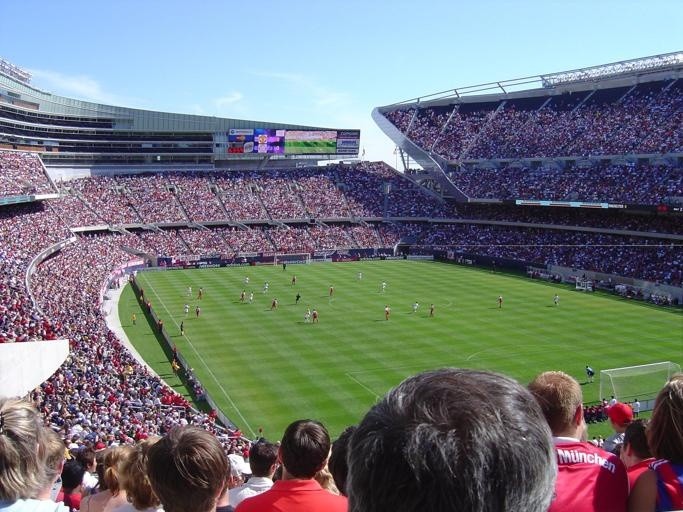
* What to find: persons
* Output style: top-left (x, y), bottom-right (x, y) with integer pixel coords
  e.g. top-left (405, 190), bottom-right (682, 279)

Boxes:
top-left (632, 397), bottom-right (642, 417)
top-left (240, 289), bottom-right (246, 301)
top-left (217, 456), bottom-right (236, 511)
top-left (328, 424), bottom-right (360, 496)
top-left (620, 418), bottom-right (657, 491)
top-left (384, 304), bottom-right (391, 320)
top-left (0, 145), bottom-right (166, 342)
top-left (584, 365), bottom-right (597, 383)
top-left (32, 344), bottom-right (250, 448)
top-left (235, 420), bottom-right (348, 512)
top-left (527, 370), bottom-right (630, 511)
top-left (271, 463), bottom-right (333, 493)
top-left (428, 303), bottom-right (435, 316)
top-left (631, 375), bottom-right (683, 511)
top-left (244, 276), bottom-right (249, 284)
top-left (295, 293), bottom-right (301, 304)
top-left (327, 285), bottom-right (334, 298)
top-left (282, 261), bottom-right (286, 270)
top-left (196, 306), bottom-right (200, 317)
top-left (263, 281), bottom-right (270, 292)
top-left (168, 161), bottom-right (455, 259)
top-left (357, 272), bottom-right (362, 280)
top-left (78, 447), bottom-right (96, 490)
top-left (114, 441), bottom-right (164, 512)
top-left (146, 424), bottom-right (226, 512)
top-left (348, 368), bottom-right (558, 512)
top-left (94, 450), bottom-right (107, 494)
top-left (291, 275), bottom-right (296, 285)
top-left (585, 397), bottom-right (619, 424)
top-left (303, 312), bottom-right (311, 322)
top-left (591, 435), bottom-right (608, 448)
top-left (248, 292), bottom-right (254, 303)
top-left (55, 459), bottom-right (81, 510)
top-left (302, 305), bottom-right (311, 315)
top-left (271, 299), bottom-right (278, 310)
top-left (187, 286), bottom-right (192, 296)
top-left (310, 309), bottom-right (318, 322)
top-left (381, 281), bottom-right (387, 291)
top-left (197, 287), bottom-right (202, 300)
top-left (29, 427), bottom-right (67, 504)
top-left (228, 440), bottom-right (278, 508)
top-left (79, 447), bottom-right (130, 512)
top-left (600, 400), bottom-right (634, 450)
top-left (181, 322), bottom-right (184, 335)
top-left (412, 301), bottom-right (418, 313)
top-left (455, 206), bottom-right (683, 314)
top-left (0, 397), bottom-right (69, 512)
top-left (184, 304), bottom-right (190, 315)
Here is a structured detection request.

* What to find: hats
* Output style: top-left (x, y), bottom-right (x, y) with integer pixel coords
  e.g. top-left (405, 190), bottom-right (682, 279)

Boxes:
top-left (606, 402), bottom-right (634, 427)
